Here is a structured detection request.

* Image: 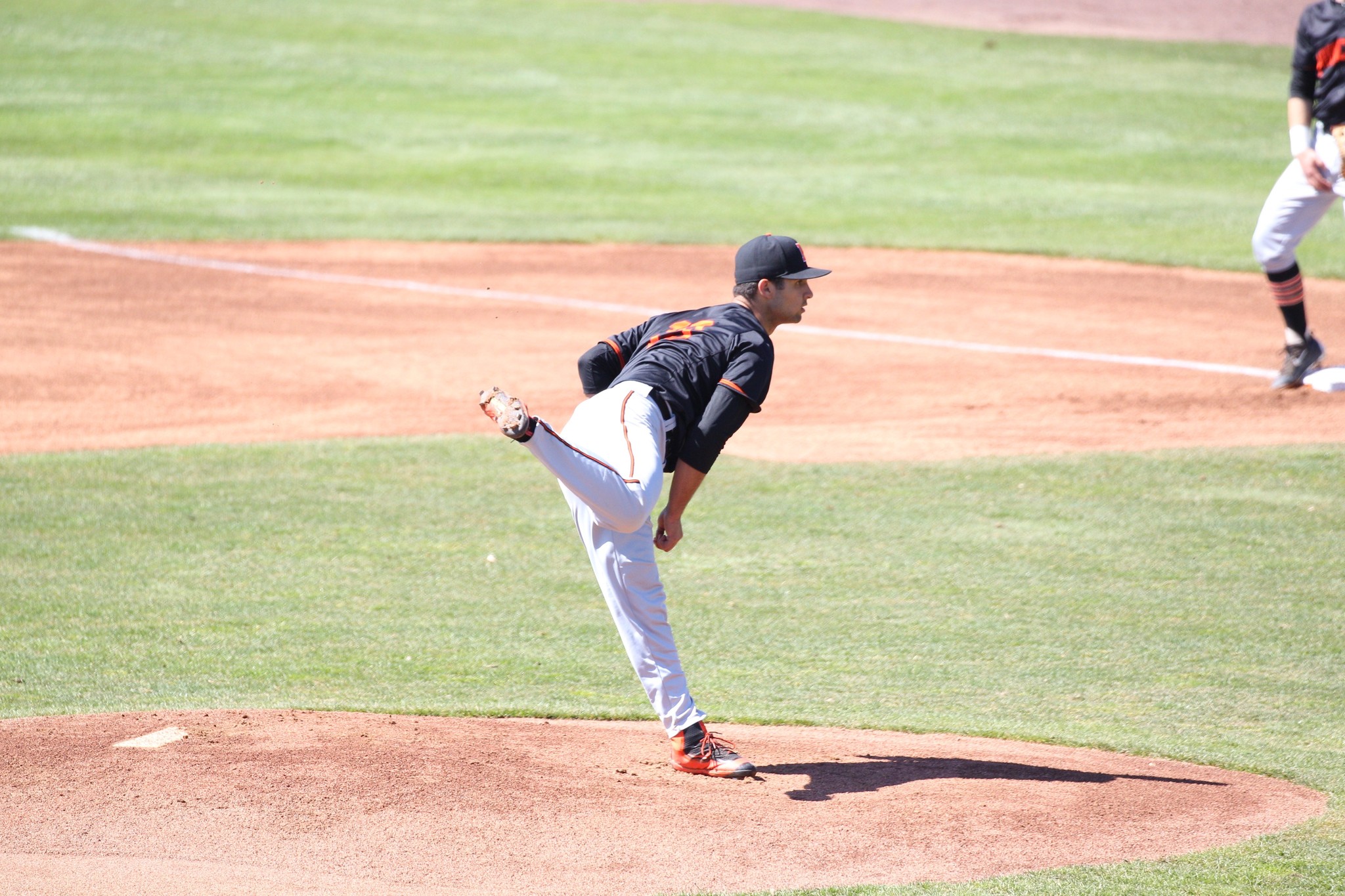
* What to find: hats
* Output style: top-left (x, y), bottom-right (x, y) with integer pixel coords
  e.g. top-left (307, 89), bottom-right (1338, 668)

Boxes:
top-left (734, 234), bottom-right (832, 286)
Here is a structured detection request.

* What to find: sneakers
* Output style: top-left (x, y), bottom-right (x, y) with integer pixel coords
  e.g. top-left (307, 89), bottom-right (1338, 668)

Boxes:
top-left (479, 387), bottom-right (530, 444)
top-left (670, 721), bottom-right (757, 779)
top-left (1270, 335), bottom-right (1324, 392)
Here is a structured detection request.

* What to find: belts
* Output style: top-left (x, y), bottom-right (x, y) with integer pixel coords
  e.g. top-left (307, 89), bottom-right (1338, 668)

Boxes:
top-left (649, 387), bottom-right (677, 473)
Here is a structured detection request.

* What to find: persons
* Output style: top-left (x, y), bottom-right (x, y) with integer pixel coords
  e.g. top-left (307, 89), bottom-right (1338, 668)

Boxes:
top-left (480, 232), bottom-right (832, 779)
top-left (1251, 0), bottom-right (1345, 391)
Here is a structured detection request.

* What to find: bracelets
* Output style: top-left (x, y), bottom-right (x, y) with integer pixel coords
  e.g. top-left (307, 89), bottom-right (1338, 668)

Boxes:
top-left (1289, 125), bottom-right (1310, 155)
